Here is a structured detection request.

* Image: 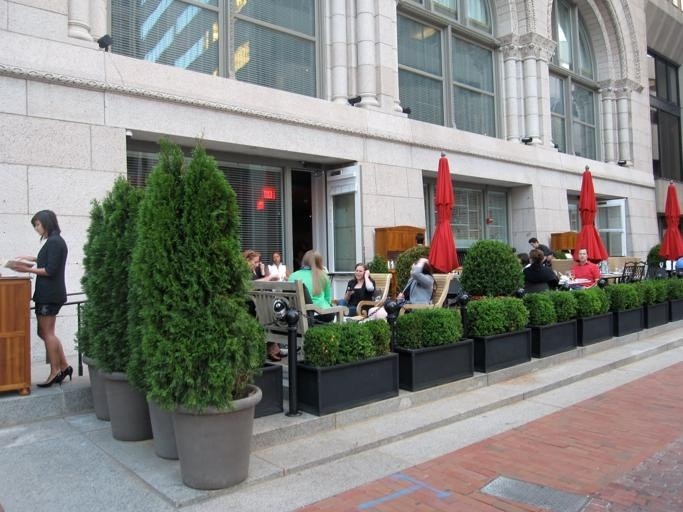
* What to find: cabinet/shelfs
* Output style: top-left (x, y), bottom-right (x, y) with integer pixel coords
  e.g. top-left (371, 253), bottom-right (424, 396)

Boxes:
top-left (0, 275), bottom-right (34, 398)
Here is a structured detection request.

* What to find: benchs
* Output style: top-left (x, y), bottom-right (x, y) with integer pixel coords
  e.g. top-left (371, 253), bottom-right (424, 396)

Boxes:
top-left (251, 273), bottom-right (451, 358)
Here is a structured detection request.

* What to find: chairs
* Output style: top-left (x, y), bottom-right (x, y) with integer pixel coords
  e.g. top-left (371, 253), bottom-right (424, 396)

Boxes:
top-left (525, 255), bottom-right (646, 291)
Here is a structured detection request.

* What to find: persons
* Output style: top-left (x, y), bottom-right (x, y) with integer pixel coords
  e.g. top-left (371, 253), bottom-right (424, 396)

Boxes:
top-left (243, 248), bottom-right (335, 361)
top-left (13, 210), bottom-right (73, 388)
top-left (512, 237), bottom-right (559, 292)
top-left (567, 248), bottom-right (601, 288)
top-left (337, 263), bottom-right (375, 317)
top-left (396, 258), bottom-right (438, 313)
top-left (415, 233), bottom-right (424, 245)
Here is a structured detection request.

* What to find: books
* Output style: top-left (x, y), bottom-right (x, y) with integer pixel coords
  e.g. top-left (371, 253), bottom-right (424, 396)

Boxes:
top-left (4, 258), bottom-right (34, 271)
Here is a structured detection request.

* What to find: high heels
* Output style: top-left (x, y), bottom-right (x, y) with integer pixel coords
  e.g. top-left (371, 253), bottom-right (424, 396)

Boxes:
top-left (62, 365), bottom-right (74, 381)
top-left (35, 369), bottom-right (64, 388)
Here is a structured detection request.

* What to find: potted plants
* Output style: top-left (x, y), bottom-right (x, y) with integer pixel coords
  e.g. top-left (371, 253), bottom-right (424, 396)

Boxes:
top-left (298, 319), bottom-right (399, 416)
top-left (395, 306), bottom-right (475, 394)
top-left (528, 271), bottom-right (682, 358)
top-left (466, 297), bottom-right (531, 374)
top-left (74, 135), bottom-right (265, 491)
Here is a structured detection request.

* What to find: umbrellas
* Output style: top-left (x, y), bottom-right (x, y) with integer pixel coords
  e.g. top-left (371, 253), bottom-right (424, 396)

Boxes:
top-left (428, 151), bottom-right (461, 272)
top-left (574, 164), bottom-right (608, 266)
top-left (659, 178), bottom-right (683, 270)
